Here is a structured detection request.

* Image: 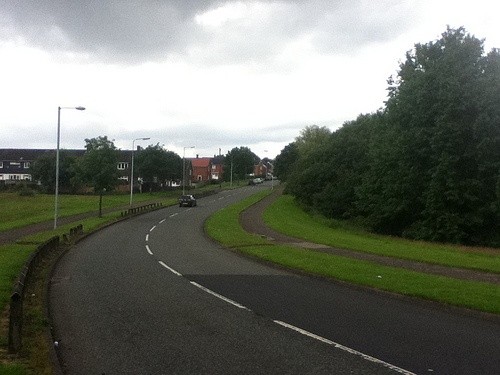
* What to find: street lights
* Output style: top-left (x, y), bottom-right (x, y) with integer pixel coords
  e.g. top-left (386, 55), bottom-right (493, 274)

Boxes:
top-left (181, 146), bottom-right (195, 196)
top-left (53, 105), bottom-right (86, 232)
top-left (129, 137), bottom-right (152, 210)
top-left (267, 161), bottom-right (274, 191)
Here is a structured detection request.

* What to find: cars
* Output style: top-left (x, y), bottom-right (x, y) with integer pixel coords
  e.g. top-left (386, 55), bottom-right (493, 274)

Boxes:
top-left (179, 195), bottom-right (196, 209)
